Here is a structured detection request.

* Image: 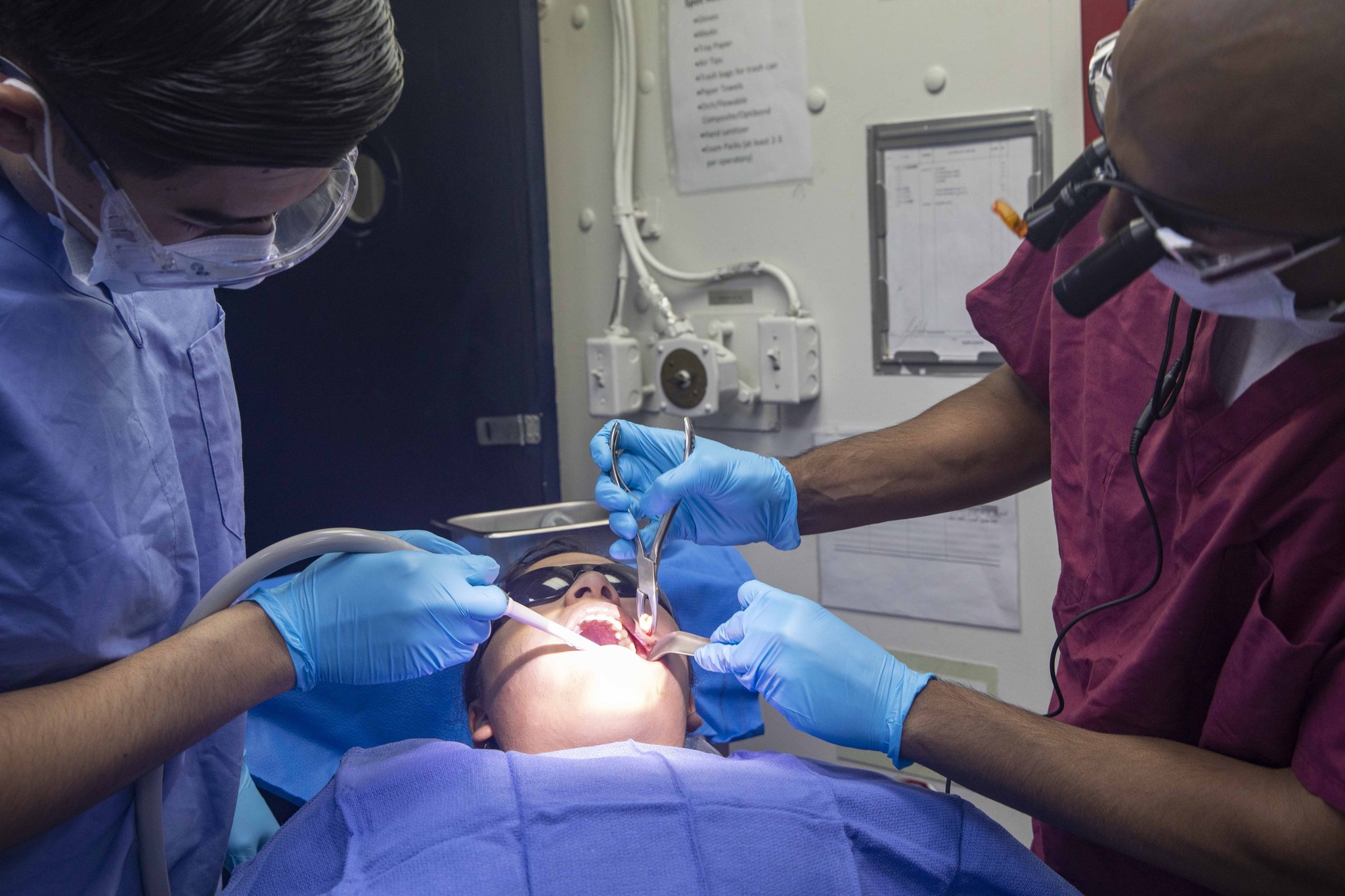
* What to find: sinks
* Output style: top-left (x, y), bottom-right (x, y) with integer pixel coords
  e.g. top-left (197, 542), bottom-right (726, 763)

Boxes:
top-left (432, 498), bottom-right (618, 580)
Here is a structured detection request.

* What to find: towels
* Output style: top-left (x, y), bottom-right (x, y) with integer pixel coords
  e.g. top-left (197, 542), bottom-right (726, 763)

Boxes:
top-left (627, 538), bottom-right (766, 743)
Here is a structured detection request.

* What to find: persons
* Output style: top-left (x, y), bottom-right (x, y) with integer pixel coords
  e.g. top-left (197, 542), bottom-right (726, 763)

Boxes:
top-left (0, 5), bottom-right (512, 895)
top-left (220, 534), bottom-right (1089, 895)
top-left (588, 1), bottom-right (1345, 893)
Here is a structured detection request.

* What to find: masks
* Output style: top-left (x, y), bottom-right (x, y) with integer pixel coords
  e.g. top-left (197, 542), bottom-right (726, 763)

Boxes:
top-left (5, 77), bottom-right (278, 295)
top-left (1149, 235), bottom-right (1345, 341)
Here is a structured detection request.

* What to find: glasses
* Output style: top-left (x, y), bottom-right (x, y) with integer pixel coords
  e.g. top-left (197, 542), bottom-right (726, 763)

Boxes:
top-left (1, 55), bottom-right (358, 289)
top-left (1090, 29), bottom-right (1344, 286)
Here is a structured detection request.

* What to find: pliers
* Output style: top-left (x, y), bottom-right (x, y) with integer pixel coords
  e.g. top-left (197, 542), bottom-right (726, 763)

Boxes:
top-left (610, 414), bottom-right (696, 634)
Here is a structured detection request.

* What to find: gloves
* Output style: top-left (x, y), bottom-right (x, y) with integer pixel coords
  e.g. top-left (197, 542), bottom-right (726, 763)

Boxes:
top-left (590, 419), bottom-right (801, 564)
top-left (694, 580), bottom-right (938, 770)
top-left (237, 530), bottom-right (508, 693)
